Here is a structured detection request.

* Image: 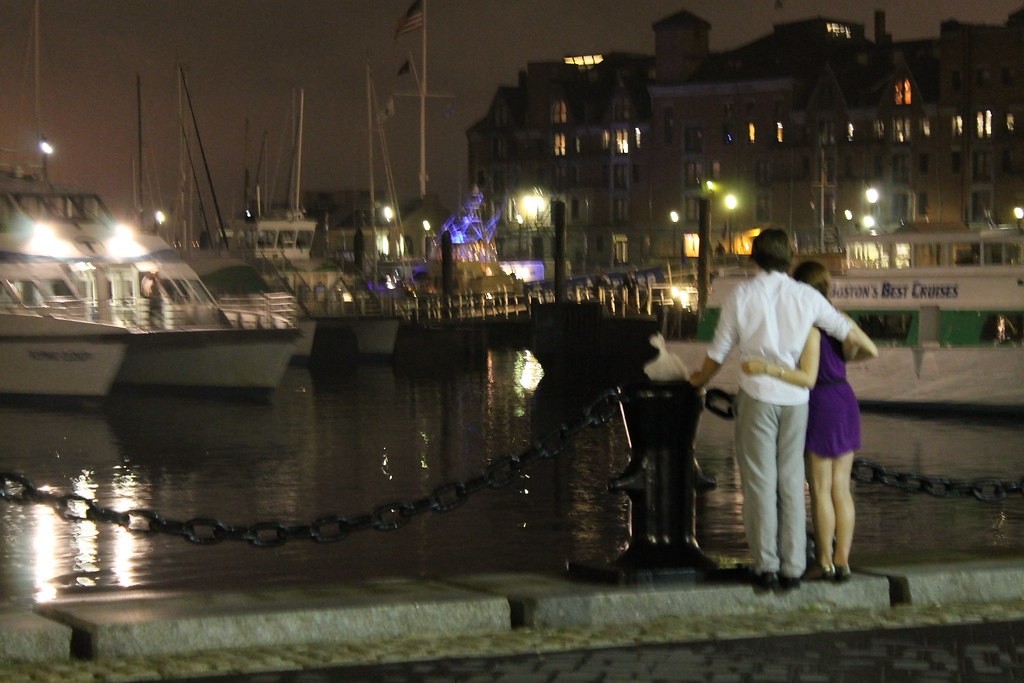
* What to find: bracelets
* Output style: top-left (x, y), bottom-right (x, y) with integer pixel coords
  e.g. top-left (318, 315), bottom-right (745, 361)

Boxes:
top-left (778, 367), bottom-right (784, 377)
top-left (765, 363), bottom-right (769, 372)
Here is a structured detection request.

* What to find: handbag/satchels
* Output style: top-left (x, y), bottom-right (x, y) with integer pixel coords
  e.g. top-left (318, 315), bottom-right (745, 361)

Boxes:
top-left (644, 334), bottom-right (694, 381)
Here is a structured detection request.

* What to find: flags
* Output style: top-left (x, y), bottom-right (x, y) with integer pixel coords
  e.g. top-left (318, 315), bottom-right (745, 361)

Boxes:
top-left (397, 62), bottom-right (409, 76)
top-left (394, 0), bottom-right (423, 39)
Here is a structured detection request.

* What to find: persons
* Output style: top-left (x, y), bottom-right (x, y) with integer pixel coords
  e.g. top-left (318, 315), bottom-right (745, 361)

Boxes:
top-left (714, 243), bottom-right (725, 256)
top-left (689, 227), bottom-right (859, 590)
top-left (741, 262), bottom-right (877, 582)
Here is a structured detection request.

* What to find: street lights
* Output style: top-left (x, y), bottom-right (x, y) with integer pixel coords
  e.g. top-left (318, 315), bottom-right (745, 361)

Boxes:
top-left (721, 190), bottom-right (740, 256)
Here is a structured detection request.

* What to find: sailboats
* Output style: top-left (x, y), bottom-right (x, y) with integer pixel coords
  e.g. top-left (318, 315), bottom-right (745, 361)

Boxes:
top-left (120, 63), bottom-right (315, 353)
top-left (1, 2), bottom-right (302, 390)
top-left (327, 0), bottom-right (529, 322)
top-left (239, 82), bottom-right (403, 353)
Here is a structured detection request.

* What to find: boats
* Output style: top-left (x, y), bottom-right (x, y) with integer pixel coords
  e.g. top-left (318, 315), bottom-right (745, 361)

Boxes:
top-left (645, 223), bottom-right (1024, 415)
top-left (0, 314), bottom-right (132, 397)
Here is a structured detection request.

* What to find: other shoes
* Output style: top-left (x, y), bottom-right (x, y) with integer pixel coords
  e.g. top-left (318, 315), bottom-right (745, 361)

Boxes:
top-left (798, 560), bottom-right (836, 581)
top-left (777, 574), bottom-right (800, 590)
top-left (835, 564), bottom-right (851, 583)
top-left (734, 566), bottom-right (775, 589)
